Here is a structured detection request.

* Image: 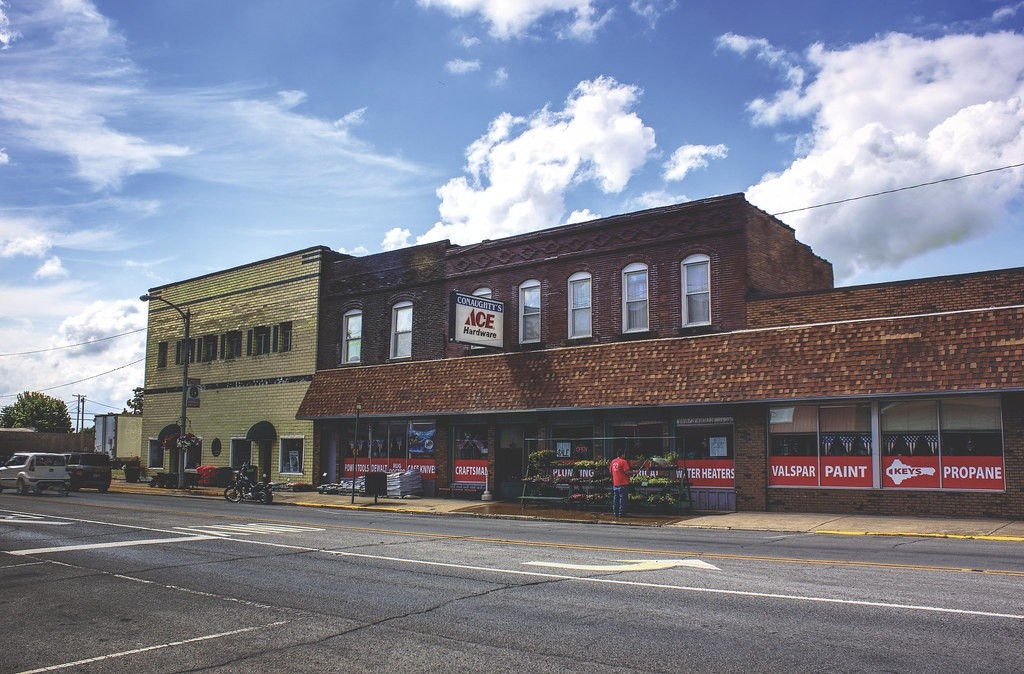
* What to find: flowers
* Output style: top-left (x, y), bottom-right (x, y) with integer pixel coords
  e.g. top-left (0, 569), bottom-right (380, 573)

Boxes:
top-left (570, 491), bottom-right (592, 499)
top-left (162, 432), bottom-right (203, 453)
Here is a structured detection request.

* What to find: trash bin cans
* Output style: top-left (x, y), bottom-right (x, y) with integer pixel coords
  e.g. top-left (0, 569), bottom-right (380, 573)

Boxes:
top-left (365, 472), bottom-right (387, 497)
top-left (124, 465), bottom-right (141, 483)
top-left (196, 465), bottom-right (258, 488)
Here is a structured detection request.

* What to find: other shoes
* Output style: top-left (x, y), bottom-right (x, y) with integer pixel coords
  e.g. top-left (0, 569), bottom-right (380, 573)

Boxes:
top-left (619, 513), bottom-right (632, 518)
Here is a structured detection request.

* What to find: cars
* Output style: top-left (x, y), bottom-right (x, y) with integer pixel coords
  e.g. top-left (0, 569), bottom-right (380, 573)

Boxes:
top-left (62, 453), bottom-right (112, 494)
top-left (0, 452), bottom-right (71, 497)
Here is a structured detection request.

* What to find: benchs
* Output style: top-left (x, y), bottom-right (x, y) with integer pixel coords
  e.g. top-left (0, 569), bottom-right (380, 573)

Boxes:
top-left (438, 487), bottom-right (479, 500)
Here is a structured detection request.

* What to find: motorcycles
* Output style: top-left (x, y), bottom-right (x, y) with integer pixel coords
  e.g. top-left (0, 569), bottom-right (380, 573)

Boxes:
top-left (224, 461), bottom-right (274, 505)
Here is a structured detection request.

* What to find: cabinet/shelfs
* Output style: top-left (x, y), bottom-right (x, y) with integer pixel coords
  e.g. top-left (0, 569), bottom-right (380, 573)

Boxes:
top-left (515, 437), bottom-right (696, 516)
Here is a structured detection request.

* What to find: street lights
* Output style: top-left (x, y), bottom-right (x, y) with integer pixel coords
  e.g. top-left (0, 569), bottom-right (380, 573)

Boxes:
top-left (140, 295), bottom-right (190, 490)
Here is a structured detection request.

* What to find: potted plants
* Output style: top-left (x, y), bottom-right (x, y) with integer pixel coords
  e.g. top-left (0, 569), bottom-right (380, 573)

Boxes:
top-left (520, 448), bottom-right (681, 506)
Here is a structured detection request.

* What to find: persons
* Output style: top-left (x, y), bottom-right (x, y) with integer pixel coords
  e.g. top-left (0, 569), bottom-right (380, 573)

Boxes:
top-left (609, 449), bottom-right (639, 518)
